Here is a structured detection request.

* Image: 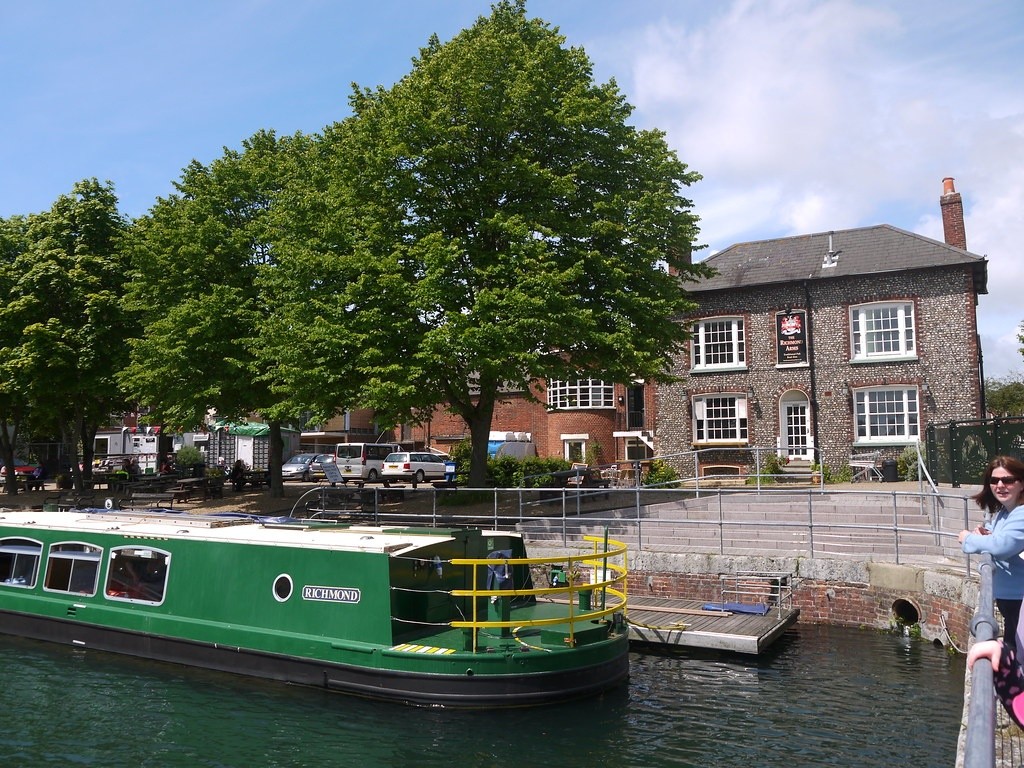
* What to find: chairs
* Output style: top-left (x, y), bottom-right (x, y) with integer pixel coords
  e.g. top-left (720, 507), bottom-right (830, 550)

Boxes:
top-left (600, 468), bottom-right (641, 487)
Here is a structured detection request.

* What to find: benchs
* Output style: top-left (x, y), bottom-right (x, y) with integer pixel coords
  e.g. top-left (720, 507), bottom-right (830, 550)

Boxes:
top-left (81, 477), bottom-right (271, 502)
top-left (540, 479), bottom-right (611, 496)
top-left (342, 485), bottom-right (406, 501)
top-left (0, 480), bottom-right (45, 493)
top-left (44, 488), bottom-right (99, 508)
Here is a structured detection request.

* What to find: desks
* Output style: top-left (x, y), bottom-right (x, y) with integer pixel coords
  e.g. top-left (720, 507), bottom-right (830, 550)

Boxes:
top-left (242, 470), bottom-right (268, 490)
top-left (2, 474), bottom-right (32, 493)
top-left (130, 473), bottom-right (175, 484)
top-left (117, 485), bottom-right (175, 508)
top-left (548, 470), bottom-right (609, 502)
top-left (348, 478), bottom-right (400, 504)
top-left (175, 476), bottom-right (210, 504)
top-left (93, 473), bottom-right (125, 478)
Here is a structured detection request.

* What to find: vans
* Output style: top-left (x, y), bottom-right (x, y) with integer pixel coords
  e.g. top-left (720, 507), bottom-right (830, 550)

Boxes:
top-left (333, 442), bottom-right (405, 483)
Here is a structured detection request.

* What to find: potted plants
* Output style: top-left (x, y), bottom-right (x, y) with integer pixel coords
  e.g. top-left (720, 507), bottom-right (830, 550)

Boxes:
top-left (810, 463), bottom-right (830, 484)
top-left (55, 473), bottom-right (74, 489)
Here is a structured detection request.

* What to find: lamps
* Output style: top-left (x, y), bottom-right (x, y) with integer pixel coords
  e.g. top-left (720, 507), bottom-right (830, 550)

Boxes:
top-left (682, 389), bottom-right (689, 403)
top-left (618, 396), bottom-right (624, 404)
top-left (842, 381), bottom-right (850, 397)
top-left (922, 377), bottom-right (928, 392)
top-left (748, 386), bottom-right (754, 398)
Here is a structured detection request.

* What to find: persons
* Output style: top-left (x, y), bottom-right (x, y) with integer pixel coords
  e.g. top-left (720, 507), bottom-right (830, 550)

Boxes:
top-left (968, 601), bottom-right (1024, 728)
top-left (21, 462), bottom-right (45, 491)
top-left (99, 461), bottom-right (105, 469)
top-left (958, 456), bottom-right (1024, 647)
top-left (123, 459), bottom-right (140, 475)
top-left (161, 460), bottom-right (174, 473)
top-left (0, 465), bottom-right (7, 480)
top-left (219, 458), bottom-right (249, 487)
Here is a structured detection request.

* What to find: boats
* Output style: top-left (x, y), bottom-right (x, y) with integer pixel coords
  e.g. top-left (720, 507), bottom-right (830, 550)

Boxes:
top-left (0, 508), bottom-right (630, 711)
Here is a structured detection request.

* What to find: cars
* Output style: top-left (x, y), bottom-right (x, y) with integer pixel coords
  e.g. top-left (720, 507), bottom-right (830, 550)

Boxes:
top-left (380, 451), bottom-right (448, 482)
top-left (0, 457), bottom-right (43, 491)
top-left (282, 454), bottom-right (335, 482)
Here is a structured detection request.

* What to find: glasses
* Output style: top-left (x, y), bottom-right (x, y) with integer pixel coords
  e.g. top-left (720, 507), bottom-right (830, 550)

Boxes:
top-left (988, 476), bottom-right (1018, 484)
